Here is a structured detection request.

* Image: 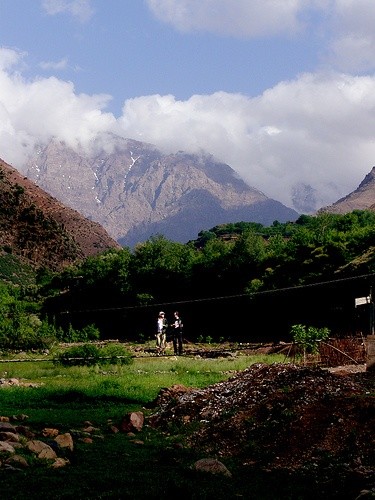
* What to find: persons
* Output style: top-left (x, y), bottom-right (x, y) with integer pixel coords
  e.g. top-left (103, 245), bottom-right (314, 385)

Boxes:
top-left (170, 311), bottom-right (184, 355)
top-left (155, 311), bottom-right (168, 354)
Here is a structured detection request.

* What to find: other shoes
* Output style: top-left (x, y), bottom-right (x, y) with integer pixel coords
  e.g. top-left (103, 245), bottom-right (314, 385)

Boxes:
top-left (162, 351), bottom-right (166, 355)
top-left (157, 352), bottom-right (160, 356)
top-left (174, 352), bottom-right (184, 356)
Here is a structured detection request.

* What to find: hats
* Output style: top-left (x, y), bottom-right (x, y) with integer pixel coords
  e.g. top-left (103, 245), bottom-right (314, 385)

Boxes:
top-left (159, 311), bottom-right (165, 315)
